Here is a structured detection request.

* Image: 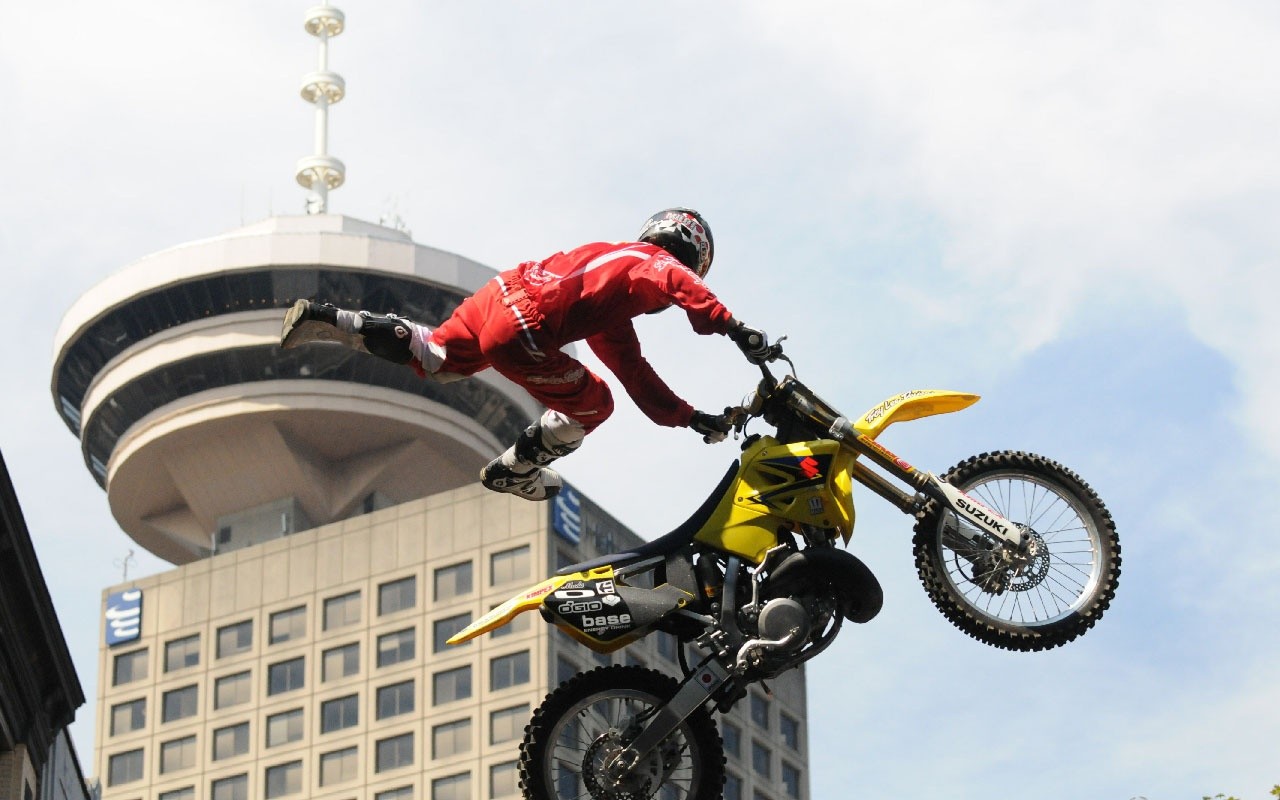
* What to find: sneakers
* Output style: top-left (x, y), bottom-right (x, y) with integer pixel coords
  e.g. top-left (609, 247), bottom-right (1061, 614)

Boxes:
top-left (480, 456), bottom-right (563, 501)
top-left (280, 299), bottom-right (337, 351)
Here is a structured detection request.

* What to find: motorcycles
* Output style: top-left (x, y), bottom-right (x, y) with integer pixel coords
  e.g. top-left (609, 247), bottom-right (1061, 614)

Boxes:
top-left (444, 334), bottom-right (1125, 799)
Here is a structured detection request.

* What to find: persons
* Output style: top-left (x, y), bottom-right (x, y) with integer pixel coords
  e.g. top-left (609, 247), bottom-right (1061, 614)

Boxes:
top-left (277, 207), bottom-right (771, 502)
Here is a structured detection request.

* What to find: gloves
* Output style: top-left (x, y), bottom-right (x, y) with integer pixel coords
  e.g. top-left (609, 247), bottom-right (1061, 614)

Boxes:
top-left (690, 410), bottom-right (732, 444)
top-left (729, 320), bottom-right (771, 366)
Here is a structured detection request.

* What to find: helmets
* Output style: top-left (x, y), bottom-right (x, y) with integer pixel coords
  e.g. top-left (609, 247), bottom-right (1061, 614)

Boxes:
top-left (638, 207), bottom-right (714, 314)
